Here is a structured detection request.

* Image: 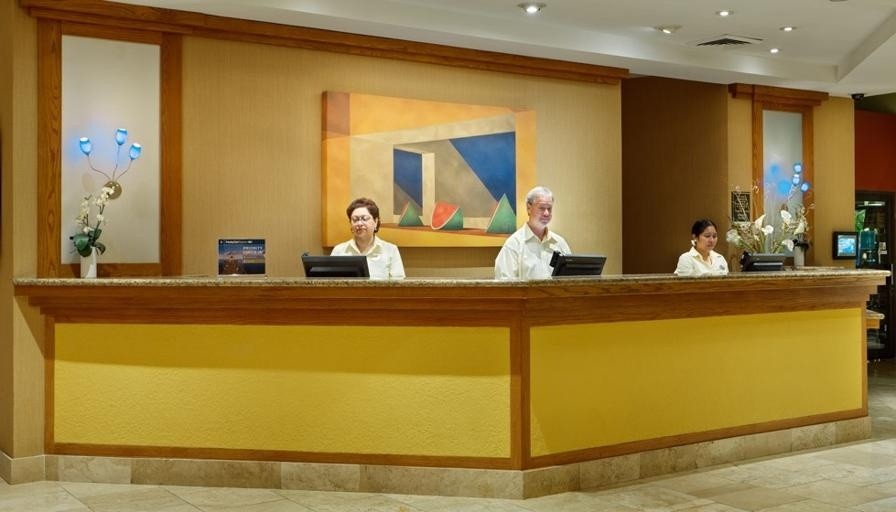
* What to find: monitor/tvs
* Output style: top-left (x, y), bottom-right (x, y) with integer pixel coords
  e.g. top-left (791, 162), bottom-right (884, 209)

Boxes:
top-left (302, 256), bottom-right (369, 277)
top-left (552, 255), bottom-right (607, 276)
top-left (832, 232), bottom-right (858, 259)
top-left (741, 254), bottom-right (786, 273)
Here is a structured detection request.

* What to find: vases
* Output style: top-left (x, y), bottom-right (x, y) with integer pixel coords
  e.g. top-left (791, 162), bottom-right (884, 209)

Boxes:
top-left (794, 246), bottom-right (804, 266)
top-left (79, 244), bottom-right (98, 279)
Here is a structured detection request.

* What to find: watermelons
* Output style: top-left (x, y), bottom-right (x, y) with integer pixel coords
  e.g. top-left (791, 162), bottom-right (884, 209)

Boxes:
top-left (398, 202), bottom-right (423, 226)
top-left (431, 201), bottom-right (464, 231)
top-left (485, 193), bottom-right (517, 234)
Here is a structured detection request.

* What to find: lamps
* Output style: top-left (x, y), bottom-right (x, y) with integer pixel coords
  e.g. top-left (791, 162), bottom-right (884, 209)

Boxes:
top-left (776, 162), bottom-right (813, 218)
top-left (76, 127), bottom-right (147, 197)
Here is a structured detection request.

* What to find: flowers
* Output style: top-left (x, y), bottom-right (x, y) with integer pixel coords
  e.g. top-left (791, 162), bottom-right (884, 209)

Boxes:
top-left (727, 174), bottom-right (818, 253)
top-left (67, 183), bottom-right (116, 257)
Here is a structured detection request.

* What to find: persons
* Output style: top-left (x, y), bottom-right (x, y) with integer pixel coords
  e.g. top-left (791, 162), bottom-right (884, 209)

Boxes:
top-left (329, 198), bottom-right (406, 278)
top-left (495, 186), bottom-right (571, 281)
top-left (674, 219), bottom-right (729, 274)
